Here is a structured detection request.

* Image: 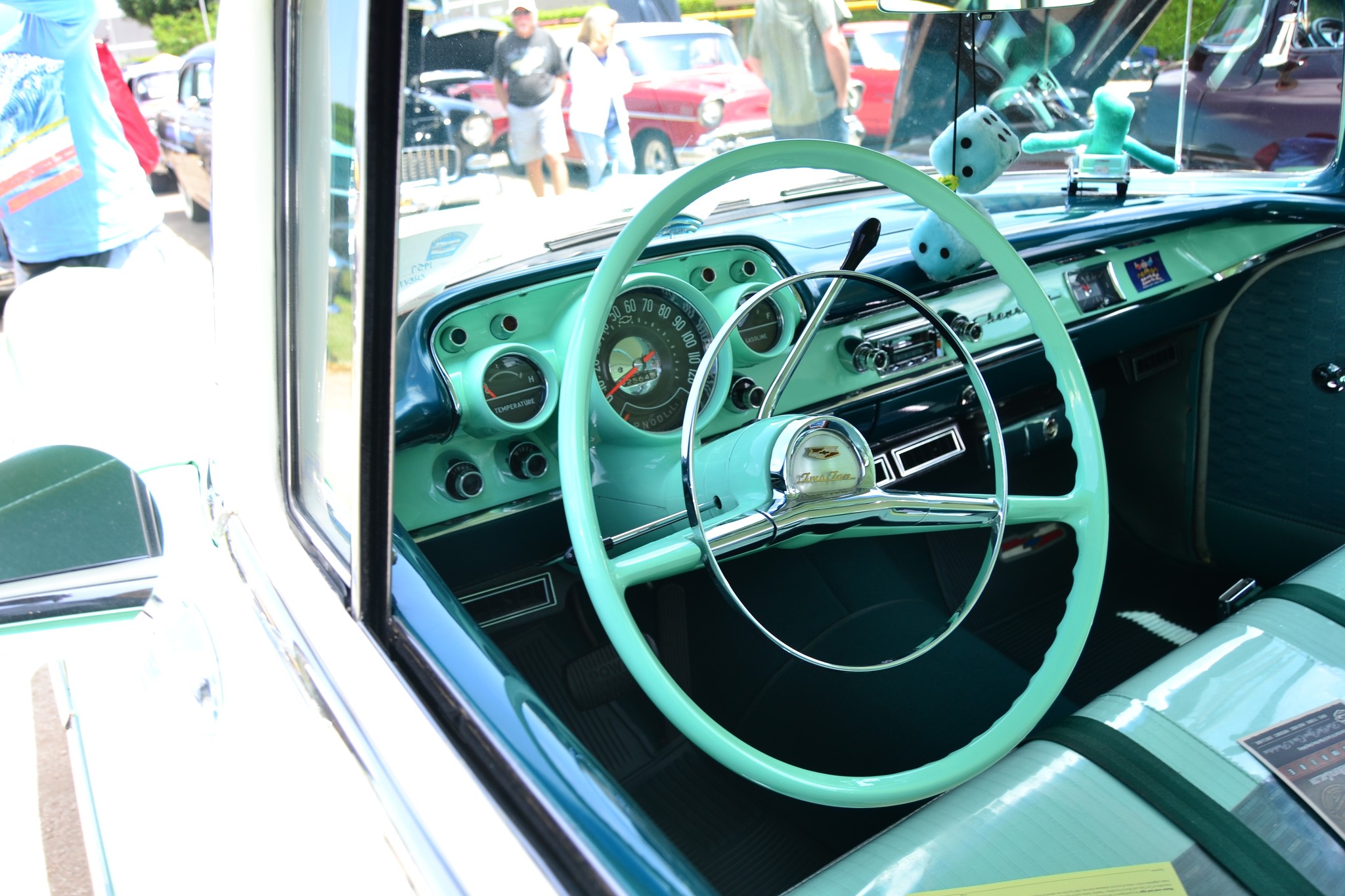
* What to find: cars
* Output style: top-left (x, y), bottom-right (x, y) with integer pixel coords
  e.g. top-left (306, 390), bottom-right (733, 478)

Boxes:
top-left (1141, 0), bottom-right (1345, 174)
top-left (117, 7), bottom-right (911, 222)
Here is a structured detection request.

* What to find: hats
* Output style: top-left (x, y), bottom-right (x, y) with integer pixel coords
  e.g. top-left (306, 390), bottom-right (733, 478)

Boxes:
top-left (507, 0), bottom-right (539, 15)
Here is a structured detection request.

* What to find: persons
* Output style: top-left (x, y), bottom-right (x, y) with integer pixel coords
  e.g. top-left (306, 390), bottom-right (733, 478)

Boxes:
top-left (0, 1), bottom-right (166, 286)
top-left (749, 0), bottom-right (855, 145)
top-left (492, 0), bottom-right (573, 197)
top-left (566, 2), bottom-right (639, 190)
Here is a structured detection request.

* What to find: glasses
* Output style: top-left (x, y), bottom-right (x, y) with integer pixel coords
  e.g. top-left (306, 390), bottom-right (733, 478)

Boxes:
top-left (513, 10), bottom-right (530, 15)
